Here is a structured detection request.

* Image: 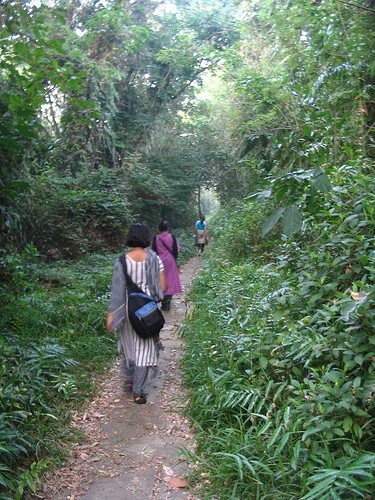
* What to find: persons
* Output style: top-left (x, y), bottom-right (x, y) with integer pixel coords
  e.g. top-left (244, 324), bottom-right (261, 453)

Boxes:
top-left (106, 226), bottom-right (166, 403)
top-left (150, 222), bottom-right (180, 311)
top-left (194, 215), bottom-right (209, 256)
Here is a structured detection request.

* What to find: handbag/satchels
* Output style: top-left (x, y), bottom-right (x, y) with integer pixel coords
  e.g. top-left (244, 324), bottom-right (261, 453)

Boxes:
top-left (126, 275), bottom-right (166, 339)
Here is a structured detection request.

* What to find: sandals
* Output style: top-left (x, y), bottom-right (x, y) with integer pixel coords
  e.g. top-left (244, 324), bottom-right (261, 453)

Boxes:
top-left (133, 393), bottom-right (146, 403)
top-left (124, 381), bottom-right (133, 392)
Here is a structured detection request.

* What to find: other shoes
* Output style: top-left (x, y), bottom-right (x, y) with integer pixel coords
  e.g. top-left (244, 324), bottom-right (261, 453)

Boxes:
top-left (161, 302), bottom-right (171, 311)
